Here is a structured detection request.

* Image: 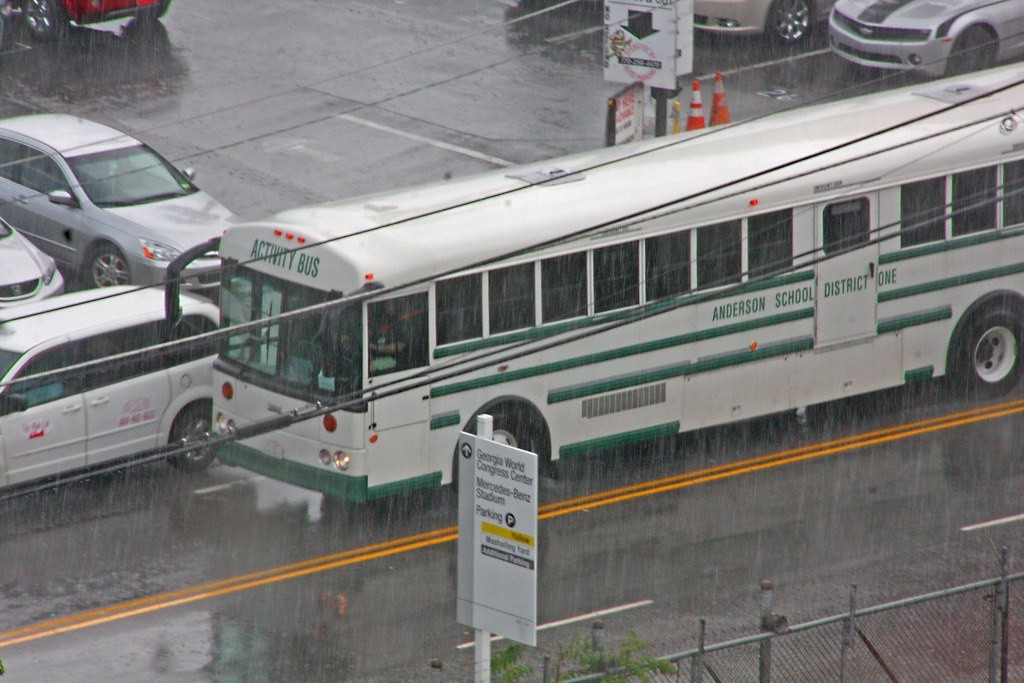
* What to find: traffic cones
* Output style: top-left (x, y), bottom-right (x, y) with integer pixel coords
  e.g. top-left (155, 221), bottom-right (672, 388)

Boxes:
top-left (708, 68), bottom-right (731, 127)
top-left (685, 79), bottom-right (706, 132)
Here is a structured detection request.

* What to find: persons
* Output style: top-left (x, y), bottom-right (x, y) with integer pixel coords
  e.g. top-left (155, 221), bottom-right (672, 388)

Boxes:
top-left (369, 313), bottom-right (412, 373)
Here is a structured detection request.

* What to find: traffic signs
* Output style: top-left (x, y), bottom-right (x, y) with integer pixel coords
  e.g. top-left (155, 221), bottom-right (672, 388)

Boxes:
top-left (603, 0), bottom-right (678, 92)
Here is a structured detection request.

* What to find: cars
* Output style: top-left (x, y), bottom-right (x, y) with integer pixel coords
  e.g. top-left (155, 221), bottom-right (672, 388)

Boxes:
top-left (0, 282), bottom-right (249, 502)
top-left (0, 0), bottom-right (171, 44)
top-left (829, 0), bottom-right (1024, 80)
top-left (692, 0), bottom-right (834, 47)
top-left (0, 109), bottom-right (242, 294)
top-left (0, 216), bottom-right (66, 312)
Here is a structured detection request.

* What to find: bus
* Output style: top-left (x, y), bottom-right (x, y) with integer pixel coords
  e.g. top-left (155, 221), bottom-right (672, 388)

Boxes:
top-left (207, 57), bottom-right (1024, 506)
